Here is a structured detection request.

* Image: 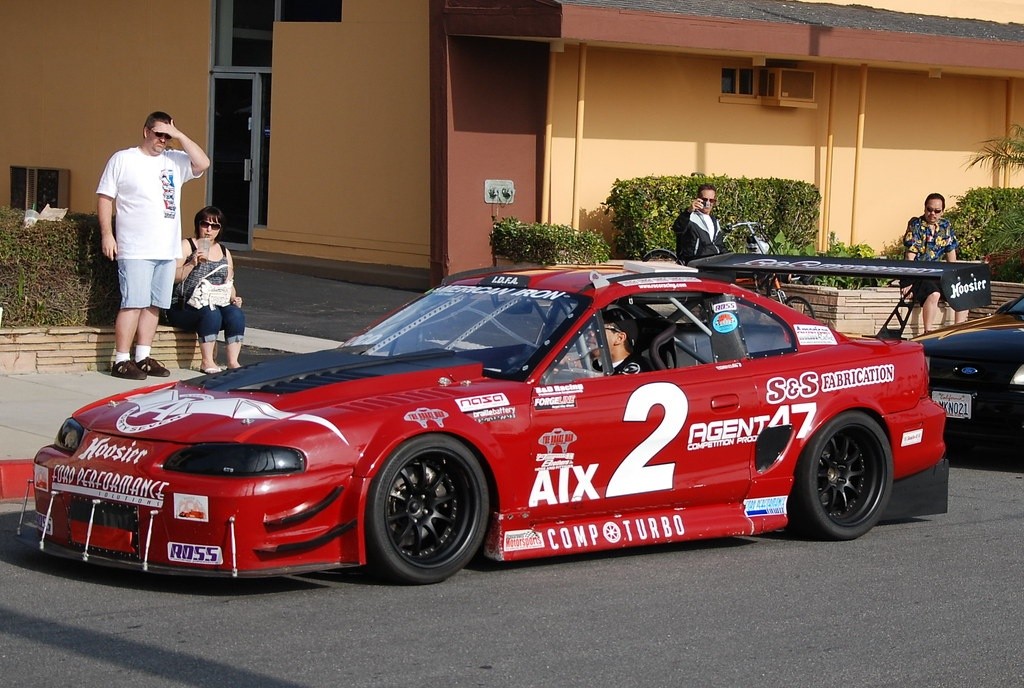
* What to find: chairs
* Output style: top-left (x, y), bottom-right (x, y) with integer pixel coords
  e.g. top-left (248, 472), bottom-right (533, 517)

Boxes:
top-left (633, 317), bottom-right (680, 371)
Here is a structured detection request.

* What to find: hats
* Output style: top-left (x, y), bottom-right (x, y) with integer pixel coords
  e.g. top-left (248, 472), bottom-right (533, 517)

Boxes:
top-left (603, 307), bottom-right (640, 348)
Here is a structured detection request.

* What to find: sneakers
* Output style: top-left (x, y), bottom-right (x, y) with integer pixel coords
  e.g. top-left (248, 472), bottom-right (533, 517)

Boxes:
top-left (132, 357), bottom-right (172, 377)
top-left (111, 359), bottom-right (147, 380)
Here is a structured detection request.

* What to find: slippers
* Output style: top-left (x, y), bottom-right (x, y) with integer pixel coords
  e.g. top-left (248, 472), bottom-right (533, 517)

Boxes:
top-left (199, 366), bottom-right (224, 374)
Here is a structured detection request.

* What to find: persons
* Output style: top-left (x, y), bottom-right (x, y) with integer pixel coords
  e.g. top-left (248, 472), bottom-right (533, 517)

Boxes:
top-left (95, 110), bottom-right (210, 380)
top-left (901, 193), bottom-right (969, 332)
top-left (672, 183), bottom-right (729, 267)
top-left (159, 206), bottom-right (246, 374)
top-left (585, 308), bottom-right (655, 375)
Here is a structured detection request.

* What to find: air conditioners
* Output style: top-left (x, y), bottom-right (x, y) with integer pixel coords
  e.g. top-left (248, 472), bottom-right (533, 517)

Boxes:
top-left (759, 66), bottom-right (816, 102)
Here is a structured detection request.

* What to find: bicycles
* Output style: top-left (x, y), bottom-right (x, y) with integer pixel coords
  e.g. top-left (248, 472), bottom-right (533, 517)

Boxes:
top-left (644, 220), bottom-right (815, 326)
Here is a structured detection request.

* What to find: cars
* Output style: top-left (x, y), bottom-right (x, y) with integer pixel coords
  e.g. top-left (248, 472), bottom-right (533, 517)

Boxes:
top-left (910, 292), bottom-right (1024, 456)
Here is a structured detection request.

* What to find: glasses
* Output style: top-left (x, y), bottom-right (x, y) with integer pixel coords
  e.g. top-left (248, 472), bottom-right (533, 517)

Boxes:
top-left (583, 325), bottom-right (622, 334)
top-left (200, 220), bottom-right (220, 231)
top-left (926, 205), bottom-right (943, 215)
top-left (149, 128), bottom-right (173, 140)
top-left (699, 197), bottom-right (716, 204)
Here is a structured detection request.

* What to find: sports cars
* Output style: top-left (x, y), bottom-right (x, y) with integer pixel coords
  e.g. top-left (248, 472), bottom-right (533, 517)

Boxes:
top-left (20, 259), bottom-right (954, 590)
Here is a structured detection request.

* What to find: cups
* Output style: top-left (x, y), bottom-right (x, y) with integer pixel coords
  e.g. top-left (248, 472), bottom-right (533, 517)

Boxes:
top-left (24, 209), bottom-right (39, 227)
top-left (197, 238), bottom-right (211, 263)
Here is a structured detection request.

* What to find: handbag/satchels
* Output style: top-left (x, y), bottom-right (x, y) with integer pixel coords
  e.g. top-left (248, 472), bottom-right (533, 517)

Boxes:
top-left (187, 264), bottom-right (233, 308)
